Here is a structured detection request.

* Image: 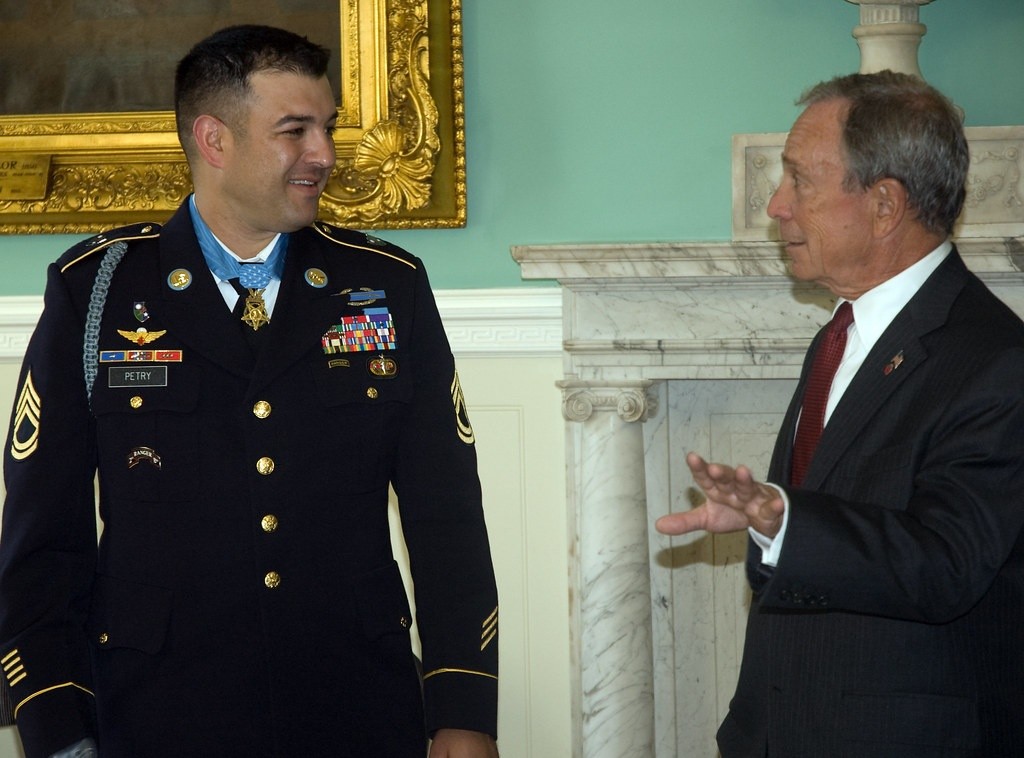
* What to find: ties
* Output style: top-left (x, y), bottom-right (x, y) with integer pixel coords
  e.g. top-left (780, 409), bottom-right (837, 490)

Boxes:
top-left (790, 301), bottom-right (854, 488)
top-left (227, 262), bottom-right (270, 361)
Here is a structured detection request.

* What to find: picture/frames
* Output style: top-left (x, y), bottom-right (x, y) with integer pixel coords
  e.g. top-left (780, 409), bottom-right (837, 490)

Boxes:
top-left (0, 0), bottom-right (468, 235)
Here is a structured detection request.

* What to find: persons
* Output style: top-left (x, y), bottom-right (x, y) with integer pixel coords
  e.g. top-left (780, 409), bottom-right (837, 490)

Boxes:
top-left (0, 25), bottom-right (498, 757)
top-left (656, 70), bottom-right (1024, 758)
